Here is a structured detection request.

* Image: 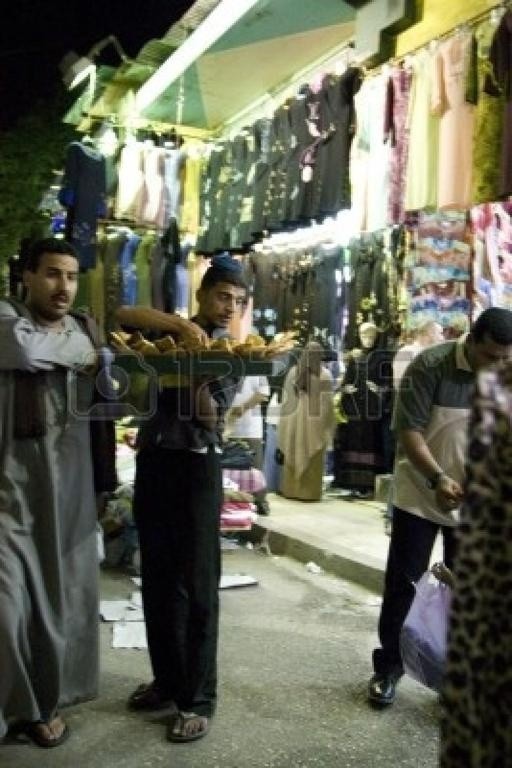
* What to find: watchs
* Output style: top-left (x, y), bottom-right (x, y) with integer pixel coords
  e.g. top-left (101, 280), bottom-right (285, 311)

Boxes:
top-left (425, 472), bottom-right (443, 490)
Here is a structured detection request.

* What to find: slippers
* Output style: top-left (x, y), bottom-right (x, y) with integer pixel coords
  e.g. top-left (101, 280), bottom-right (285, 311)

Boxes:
top-left (19, 711), bottom-right (70, 749)
top-left (127, 682), bottom-right (164, 709)
top-left (168, 710), bottom-right (210, 742)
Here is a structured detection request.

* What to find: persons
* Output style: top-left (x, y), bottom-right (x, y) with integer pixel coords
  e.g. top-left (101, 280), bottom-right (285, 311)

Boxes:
top-left (367, 307), bottom-right (512, 705)
top-left (107, 256), bottom-right (247, 741)
top-left (431, 561), bottom-right (454, 587)
top-left (216, 334), bottom-right (271, 515)
top-left (276, 342), bottom-right (335, 502)
top-left (337, 322), bottom-right (393, 499)
top-left (262, 333), bottom-right (307, 491)
top-left (1, 239), bottom-right (112, 749)
top-left (392, 320), bottom-right (443, 394)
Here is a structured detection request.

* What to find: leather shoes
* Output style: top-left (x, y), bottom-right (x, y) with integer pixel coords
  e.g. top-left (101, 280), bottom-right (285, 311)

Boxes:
top-left (367, 673), bottom-right (400, 706)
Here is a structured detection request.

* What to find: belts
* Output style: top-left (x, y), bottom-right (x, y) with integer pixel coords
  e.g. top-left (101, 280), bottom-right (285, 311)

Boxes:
top-left (194, 443), bottom-right (224, 455)
